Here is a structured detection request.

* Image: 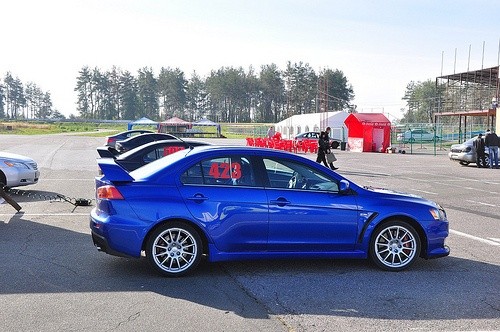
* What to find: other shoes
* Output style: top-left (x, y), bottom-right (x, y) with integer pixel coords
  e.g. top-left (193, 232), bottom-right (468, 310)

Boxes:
top-left (483, 165), bottom-right (489, 168)
top-left (477, 165), bottom-right (482, 168)
top-left (332, 168), bottom-right (338, 170)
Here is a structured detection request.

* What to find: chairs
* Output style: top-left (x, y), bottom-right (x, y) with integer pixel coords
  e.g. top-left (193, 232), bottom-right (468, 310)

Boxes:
top-left (246, 137), bottom-right (318, 154)
top-left (234, 163), bottom-right (251, 186)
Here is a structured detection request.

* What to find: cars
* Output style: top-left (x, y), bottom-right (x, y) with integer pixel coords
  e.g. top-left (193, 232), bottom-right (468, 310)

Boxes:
top-left (97, 138), bottom-right (250, 175)
top-left (293, 131), bottom-right (341, 149)
top-left (106, 129), bottom-right (154, 147)
top-left (114, 133), bottom-right (179, 155)
top-left (448, 136), bottom-right (500, 168)
top-left (0, 151), bottom-right (40, 189)
top-left (396, 128), bottom-right (441, 144)
top-left (89, 145), bottom-right (449, 277)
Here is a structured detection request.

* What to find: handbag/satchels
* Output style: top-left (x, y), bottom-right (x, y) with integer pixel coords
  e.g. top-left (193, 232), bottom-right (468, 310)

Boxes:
top-left (323, 149), bottom-right (337, 164)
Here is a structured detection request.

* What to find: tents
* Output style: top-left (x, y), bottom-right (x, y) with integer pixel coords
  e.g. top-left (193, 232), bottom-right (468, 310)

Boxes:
top-left (343, 112), bottom-right (391, 153)
top-left (274, 110), bottom-right (350, 149)
top-left (191, 118), bottom-right (221, 138)
top-left (128, 116), bottom-right (159, 130)
top-left (158, 117), bottom-right (192, 133)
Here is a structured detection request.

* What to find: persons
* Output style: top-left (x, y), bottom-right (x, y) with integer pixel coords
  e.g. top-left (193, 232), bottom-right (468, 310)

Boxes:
top-left (316, 126), bottom-right (339, 171)
top-left (475, 133), bottom-right (489, 168)
top-left (485, 129), bottom-right (499, 169)
top-left (266, 126), bottom-right (274, 140)
